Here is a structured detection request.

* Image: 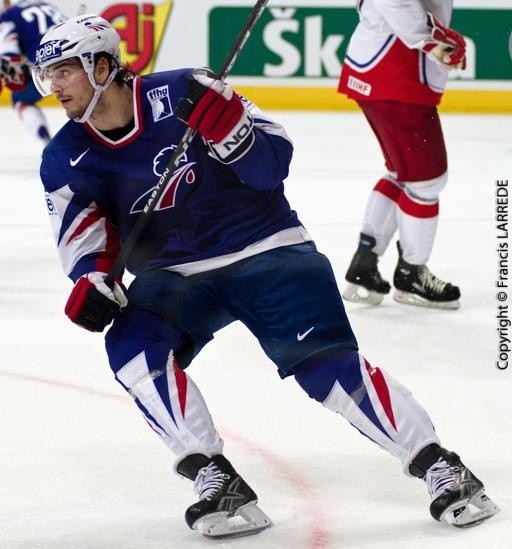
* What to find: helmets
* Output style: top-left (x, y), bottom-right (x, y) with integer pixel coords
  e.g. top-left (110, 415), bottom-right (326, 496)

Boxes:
top-left (32, 14), bottom-right (120, 97)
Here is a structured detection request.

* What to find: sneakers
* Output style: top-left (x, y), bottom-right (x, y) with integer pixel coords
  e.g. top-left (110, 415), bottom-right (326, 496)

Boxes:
top-left (177, 453), bottom-right (257, 530)
top-left (393, 241), bottom-right (460, 302)
top-left (409, 443), bottom-right (484, 520)
top-left (345, 232), bottom-right (390, 294)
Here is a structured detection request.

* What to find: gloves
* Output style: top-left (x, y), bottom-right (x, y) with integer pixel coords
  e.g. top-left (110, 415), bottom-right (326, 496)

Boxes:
top-left (65, 272), bottom-right (128, 332)
top-left (175, 68), bottom-right (255, 165)
top-left (423, 13), bottom-right (466, 70)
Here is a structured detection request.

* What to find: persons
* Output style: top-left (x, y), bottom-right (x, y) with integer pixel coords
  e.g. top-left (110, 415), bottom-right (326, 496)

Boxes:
top-left (0, 1), bottom-right (71, 142)
top-left (337, 1), bottom-right (468, 309)
top-left (29, 13), bottom-right (501, 538)
